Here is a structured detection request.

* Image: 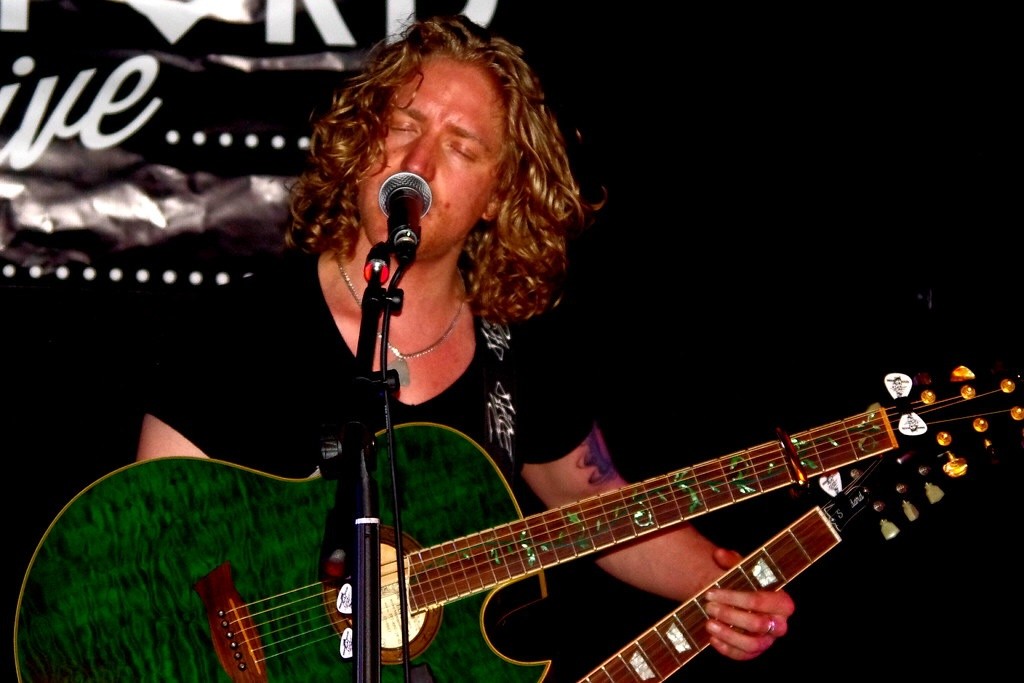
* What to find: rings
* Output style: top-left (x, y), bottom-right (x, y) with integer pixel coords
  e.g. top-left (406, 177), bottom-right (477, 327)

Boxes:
top-left (766, 619), bottom-right (774, 634)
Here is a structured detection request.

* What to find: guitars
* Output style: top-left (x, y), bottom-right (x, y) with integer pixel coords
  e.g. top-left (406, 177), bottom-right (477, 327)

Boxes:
top-left (10, 363), bottom-right (1024, 683)
top-left (578, 428), bottom-right (972, 681)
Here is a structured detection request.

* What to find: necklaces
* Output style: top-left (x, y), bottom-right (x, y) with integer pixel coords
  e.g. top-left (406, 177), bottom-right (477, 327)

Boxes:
top-left (334, 253), bottom-right (466, 385)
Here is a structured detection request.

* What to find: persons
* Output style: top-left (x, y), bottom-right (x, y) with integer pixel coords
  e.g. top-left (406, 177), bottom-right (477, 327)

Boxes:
top-left (135, 14), bottom-right (796, 661)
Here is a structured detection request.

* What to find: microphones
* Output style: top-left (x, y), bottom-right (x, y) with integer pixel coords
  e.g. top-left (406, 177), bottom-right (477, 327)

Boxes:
top-left (378, 171), bottom-right (433, 266)
